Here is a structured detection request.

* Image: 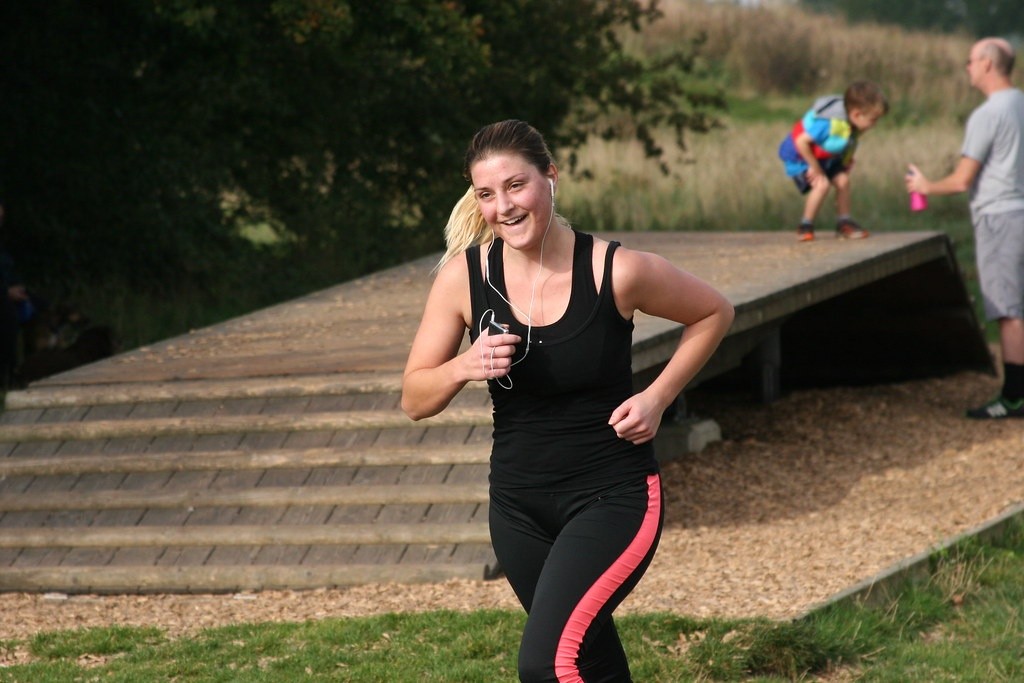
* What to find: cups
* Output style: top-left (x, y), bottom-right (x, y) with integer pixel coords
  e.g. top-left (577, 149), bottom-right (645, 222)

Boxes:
top-left (910, 192), bottom-right (927, 212)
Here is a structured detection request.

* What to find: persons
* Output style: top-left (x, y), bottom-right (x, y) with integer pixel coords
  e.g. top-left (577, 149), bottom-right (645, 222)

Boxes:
top-left (904, 35), bottom-right (1024, 422)
top-left (398, 120), bottom-right (737, 683)
top-left (775, 80), bottom-right (891, 240)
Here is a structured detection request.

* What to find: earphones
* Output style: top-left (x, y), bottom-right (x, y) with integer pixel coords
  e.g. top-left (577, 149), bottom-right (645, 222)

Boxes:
top-left (547, 178), bottom-right (555, 199)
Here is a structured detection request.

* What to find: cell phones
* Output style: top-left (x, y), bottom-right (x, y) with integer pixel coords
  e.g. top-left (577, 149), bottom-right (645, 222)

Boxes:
top-left (488, 321), bottom-right (509, 336)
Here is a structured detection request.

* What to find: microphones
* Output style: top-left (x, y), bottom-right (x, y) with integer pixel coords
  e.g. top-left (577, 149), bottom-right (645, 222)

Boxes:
top-left (485, 258), bottom-right (489, 278)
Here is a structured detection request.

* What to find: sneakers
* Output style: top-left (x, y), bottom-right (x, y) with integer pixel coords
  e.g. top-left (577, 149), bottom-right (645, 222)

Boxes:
top-left (965, 395), bottom-right (1024, 419)
top-left (797, 223), bottom-right (814, 242)
top-left (835, 221), bottom-right (869, 239)
